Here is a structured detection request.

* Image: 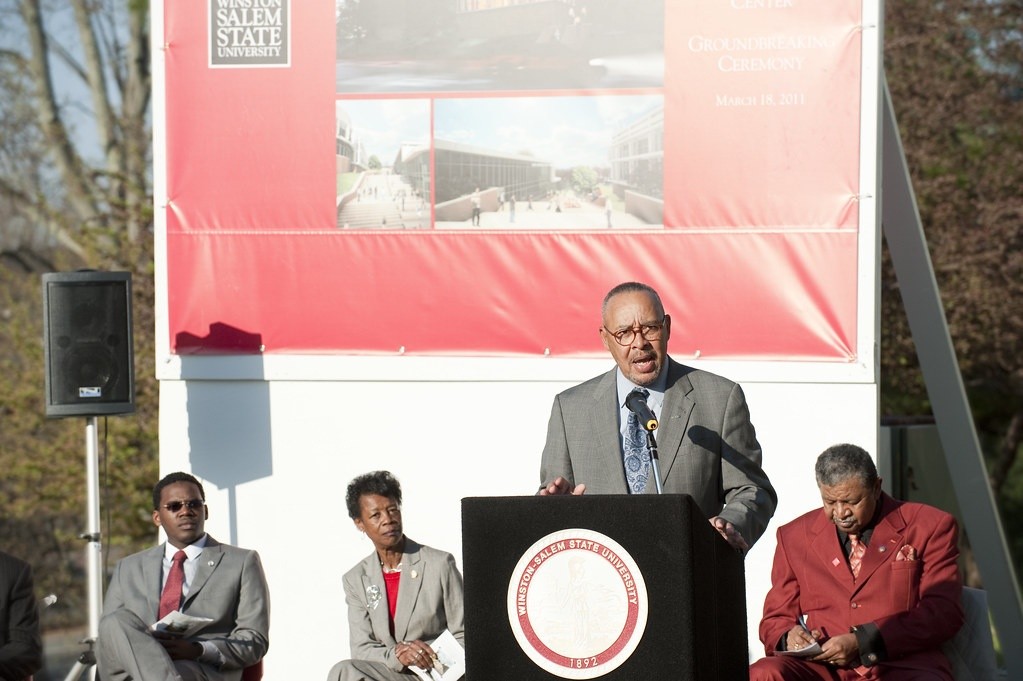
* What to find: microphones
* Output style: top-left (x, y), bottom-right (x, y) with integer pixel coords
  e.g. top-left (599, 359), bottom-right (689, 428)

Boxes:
top-left (626, 391), bottom-right (658, 431)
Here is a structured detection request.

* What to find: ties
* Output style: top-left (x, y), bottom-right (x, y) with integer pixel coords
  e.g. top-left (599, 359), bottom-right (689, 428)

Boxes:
top-left (847, 532), bottom-right (867, 584)
top-left (159, 550), bottom-right (188, 621)
top-left (620, 386), bottom-right (650, 494)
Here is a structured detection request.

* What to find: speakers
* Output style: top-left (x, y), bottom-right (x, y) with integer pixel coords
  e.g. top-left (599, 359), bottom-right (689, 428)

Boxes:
top-left (41, 271), bottom-right (136, 420)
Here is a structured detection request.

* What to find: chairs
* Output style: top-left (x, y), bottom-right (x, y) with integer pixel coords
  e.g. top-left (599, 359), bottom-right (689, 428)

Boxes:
top-left (941, 585), bottom-right (998, 681)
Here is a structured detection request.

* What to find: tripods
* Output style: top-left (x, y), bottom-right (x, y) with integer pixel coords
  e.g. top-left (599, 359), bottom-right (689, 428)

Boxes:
top-left (65, 415), bottom-right (104, 681)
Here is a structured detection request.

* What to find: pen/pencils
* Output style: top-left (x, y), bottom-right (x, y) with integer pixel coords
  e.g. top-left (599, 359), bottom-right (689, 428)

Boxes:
top-left (798, 616), bottom-right (810, 636)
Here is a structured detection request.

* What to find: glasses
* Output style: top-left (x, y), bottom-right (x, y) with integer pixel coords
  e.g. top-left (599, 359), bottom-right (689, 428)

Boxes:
top-left (156, 498), bottom-right (204, 513)
top-left (603, 315), bottom-right (667, 346)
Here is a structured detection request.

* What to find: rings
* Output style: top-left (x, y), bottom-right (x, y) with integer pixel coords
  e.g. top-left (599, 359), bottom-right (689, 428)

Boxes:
top-left (418, 650), bottom-right (422, 654)
top-left (830, 661), bottom-right (834, 665)
top-left (794, 644), bottom-right (798, 648)
top-left (415, 654), bottom-right (419, 658)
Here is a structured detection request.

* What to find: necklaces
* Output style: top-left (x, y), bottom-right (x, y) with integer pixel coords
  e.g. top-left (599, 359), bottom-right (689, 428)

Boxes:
top-left (381, 562), bottom-right (402, 573)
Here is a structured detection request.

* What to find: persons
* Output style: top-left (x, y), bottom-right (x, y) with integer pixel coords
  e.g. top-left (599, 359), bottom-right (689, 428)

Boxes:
top-left (341, 186), bottom-right (612, 229)
top-left (749, 444), bottom-right (967, 681)
top-left (93, 472), bottom-right (271, 681)
top-left (536, 282), bottom-right (778, 554)
top-left (0, 552), bottom-right (44, 681)
top-left (327, 470), bottom-right (467, 681)
